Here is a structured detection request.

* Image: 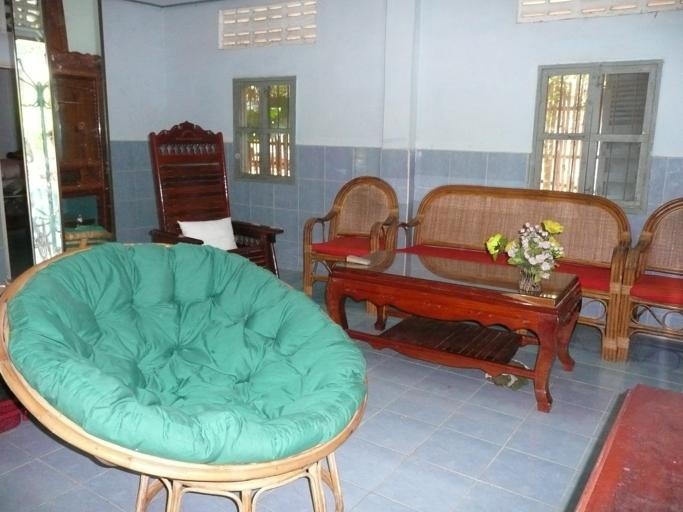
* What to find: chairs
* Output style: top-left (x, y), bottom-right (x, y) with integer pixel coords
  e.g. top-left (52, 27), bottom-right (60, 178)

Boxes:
top-left (149, 121), bottom-right (285, 280)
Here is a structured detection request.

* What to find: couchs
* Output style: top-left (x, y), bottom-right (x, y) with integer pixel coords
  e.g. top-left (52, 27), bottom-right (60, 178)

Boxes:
top-left (385, 184), bottom-right (632, 361)
top-left (617, 198), bottom-right (683, 366)
top-left (303, 177), bottom-right (399, 315)
top-left (0, 244), bottom-right (368, 512)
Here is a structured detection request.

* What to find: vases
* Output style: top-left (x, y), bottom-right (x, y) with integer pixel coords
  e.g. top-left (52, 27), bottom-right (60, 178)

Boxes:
top-left (520, 269), bottom-right (541, 291)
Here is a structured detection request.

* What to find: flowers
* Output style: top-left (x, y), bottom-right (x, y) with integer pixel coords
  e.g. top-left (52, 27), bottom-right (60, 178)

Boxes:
top-left (485, 219), bottom-right (566, 282)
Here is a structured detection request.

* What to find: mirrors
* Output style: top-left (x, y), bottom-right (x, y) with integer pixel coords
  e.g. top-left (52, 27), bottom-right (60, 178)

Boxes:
top-left (12, 39), bottom-right (61, 261)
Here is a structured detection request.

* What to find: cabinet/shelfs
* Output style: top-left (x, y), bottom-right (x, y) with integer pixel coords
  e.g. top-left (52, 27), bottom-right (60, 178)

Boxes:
top-left (48, 50), bottom-right (115, 255)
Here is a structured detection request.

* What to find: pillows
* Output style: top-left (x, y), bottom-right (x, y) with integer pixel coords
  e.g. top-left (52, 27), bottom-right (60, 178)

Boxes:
top-left (175, 217), bottom-right (238, 254)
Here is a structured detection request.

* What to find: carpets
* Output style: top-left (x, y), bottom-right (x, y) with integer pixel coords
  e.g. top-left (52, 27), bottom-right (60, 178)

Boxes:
top-left (573, 384), bottom-right (683, 511)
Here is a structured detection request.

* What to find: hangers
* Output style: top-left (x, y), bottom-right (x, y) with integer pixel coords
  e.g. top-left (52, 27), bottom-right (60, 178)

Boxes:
top-left (17, 58), bottom-right (61, 252)
